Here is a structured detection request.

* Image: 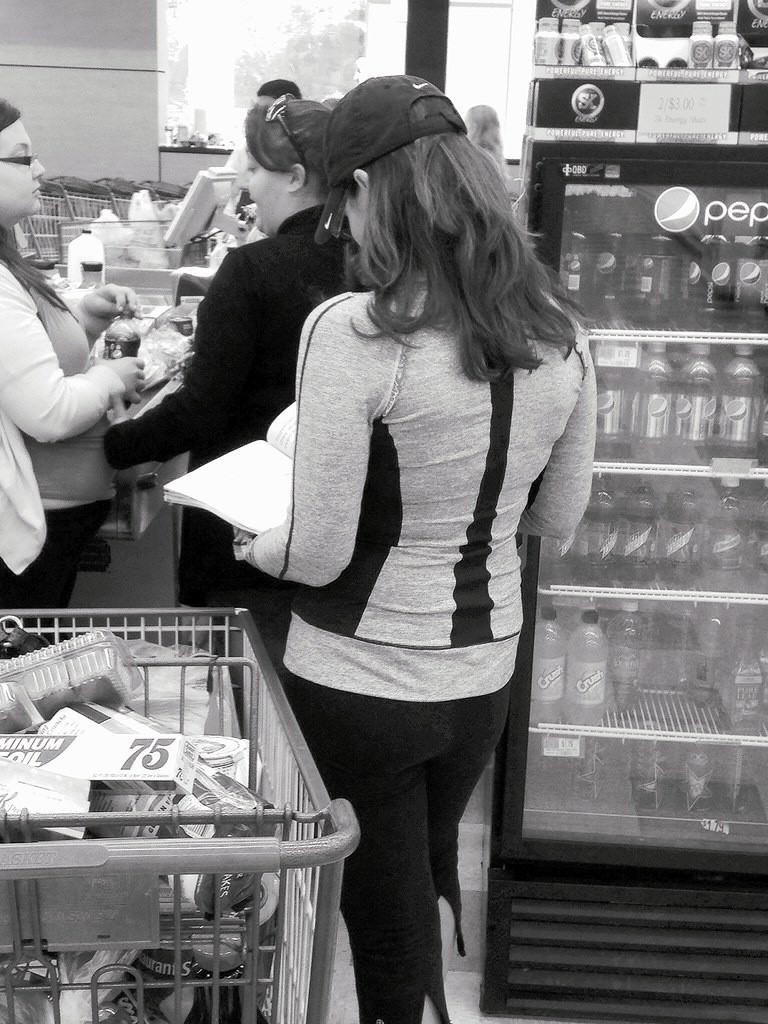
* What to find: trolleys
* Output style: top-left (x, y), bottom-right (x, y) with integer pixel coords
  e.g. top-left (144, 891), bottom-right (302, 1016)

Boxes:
top-left (0, 605), bottom-right (362, 1024)
top-left (8, 177), bottom-right (215, 273)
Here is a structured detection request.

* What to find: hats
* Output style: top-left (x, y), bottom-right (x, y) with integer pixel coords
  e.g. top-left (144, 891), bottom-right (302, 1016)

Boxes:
top-left (314, 75), bottom-right (468, 244)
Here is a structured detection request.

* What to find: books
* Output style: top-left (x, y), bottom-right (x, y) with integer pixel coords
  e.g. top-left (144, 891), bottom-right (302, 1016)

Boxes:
top-left (163, 401), bottom-right (296, 536)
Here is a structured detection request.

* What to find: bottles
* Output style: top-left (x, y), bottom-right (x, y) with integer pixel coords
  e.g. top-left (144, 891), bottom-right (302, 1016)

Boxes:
top-left (67, 229), bottom-right (105, 290)
top-left (531, 346), bottom-right (768, 713)
top-left (537, 16), bottom-right (743, 73)
top-left (104, 304), bottom-right (141, 411)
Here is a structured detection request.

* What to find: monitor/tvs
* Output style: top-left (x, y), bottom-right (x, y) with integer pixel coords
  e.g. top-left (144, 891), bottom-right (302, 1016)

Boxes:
top-left (164, 167), bottom-right (239, 245)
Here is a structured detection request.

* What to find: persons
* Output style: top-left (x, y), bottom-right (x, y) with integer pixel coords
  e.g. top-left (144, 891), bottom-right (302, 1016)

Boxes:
top-left (224, 79), bottom-right (301, 198)
top-left (464, 104), bottom-right (508, 192)
top-left (0, 99), bottom-right (145, 659)
top-left (104, 95), bottom-right (371, 695)
top-left (233, 79), bottom-right (597, 1024)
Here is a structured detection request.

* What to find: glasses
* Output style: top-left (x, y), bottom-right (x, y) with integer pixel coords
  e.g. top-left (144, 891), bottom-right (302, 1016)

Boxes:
top-left (0, 152), bottom-right (39, 169)
top-left (265, 92), bottom-right (310, 188)
